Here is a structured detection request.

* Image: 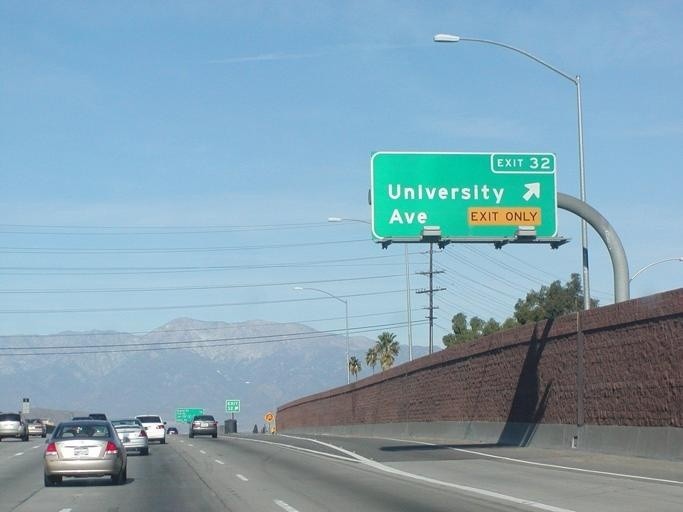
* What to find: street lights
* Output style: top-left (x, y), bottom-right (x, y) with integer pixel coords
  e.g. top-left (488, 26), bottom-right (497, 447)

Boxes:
top-left (292, 287), bottom-right (350, 384)
top-left (433, 34), bottom-right (591, 311)
top-left (328, 217), bottom-right (413, 361)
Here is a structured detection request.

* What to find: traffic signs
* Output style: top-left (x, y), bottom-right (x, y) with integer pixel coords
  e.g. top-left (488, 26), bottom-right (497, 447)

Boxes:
top-left (176, 408), bottom-right (203, 423)
top-left (226, 400), bottom-right (240, 412)
top-left (370, 151), bottom-right (557, 239)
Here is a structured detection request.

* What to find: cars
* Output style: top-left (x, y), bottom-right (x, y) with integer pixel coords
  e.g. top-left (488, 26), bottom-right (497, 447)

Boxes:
top-left (0, 413), bottom-right (46, 442)
top-left (189, 415), bottom-right (218, 438)
top-left (167, 427), bottom-right (178, 435)
top-left (42, 413), bottom-right (167, 487)
